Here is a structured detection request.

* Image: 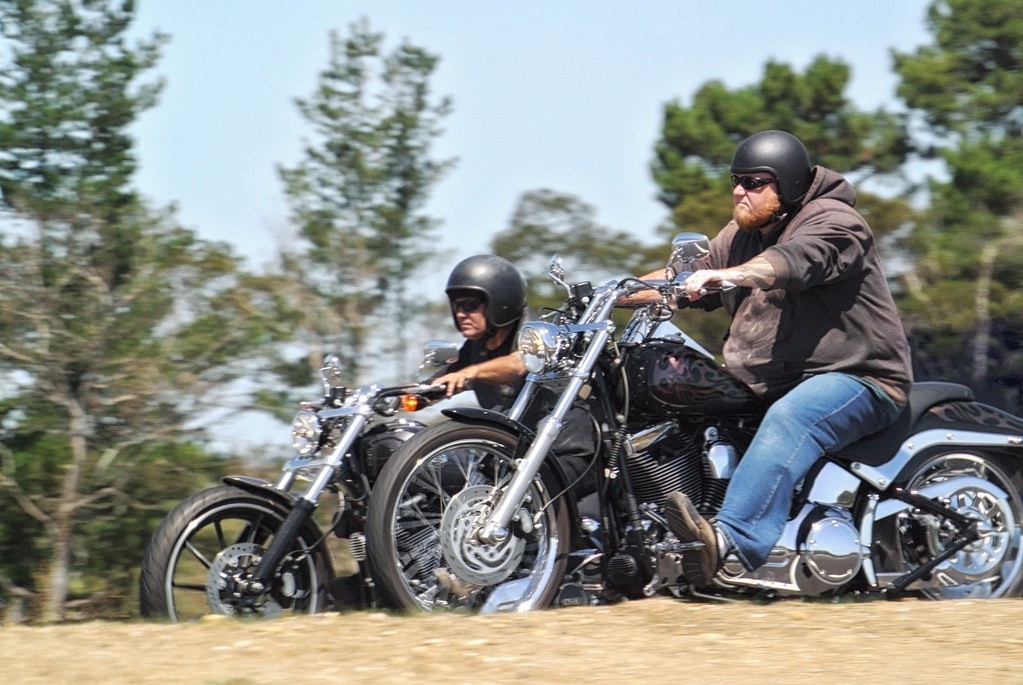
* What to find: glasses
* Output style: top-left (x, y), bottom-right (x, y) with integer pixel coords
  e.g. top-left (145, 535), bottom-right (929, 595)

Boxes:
top-left (731, 175), bottom-right (775, 190)
top-left (452, 297), bottom-right (485, 312)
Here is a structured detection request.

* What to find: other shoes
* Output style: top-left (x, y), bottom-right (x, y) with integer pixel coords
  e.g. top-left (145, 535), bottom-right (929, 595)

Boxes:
top-left (666, 491), bottom-right (720, 587)
top-left (430, 566), bottom-right (472, 600)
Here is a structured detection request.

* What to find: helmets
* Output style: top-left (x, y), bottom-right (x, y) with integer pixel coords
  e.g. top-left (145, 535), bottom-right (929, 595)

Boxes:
top-left (731, 130), bottom-right (811, 204)
top-left (445, 254), bottom-right (528, 328)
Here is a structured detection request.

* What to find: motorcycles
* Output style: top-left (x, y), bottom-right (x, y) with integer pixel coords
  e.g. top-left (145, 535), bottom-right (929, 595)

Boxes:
top-left (364, 231), bottom-right (1023, 616)
top-left (138, 339), bottom-right (472, 621)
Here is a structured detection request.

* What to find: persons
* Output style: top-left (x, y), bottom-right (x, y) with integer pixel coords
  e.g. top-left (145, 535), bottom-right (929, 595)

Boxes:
top-left (428, 253), bottom-right (600, 592)
top-left (615, 129), bottom-right (913, 586)
